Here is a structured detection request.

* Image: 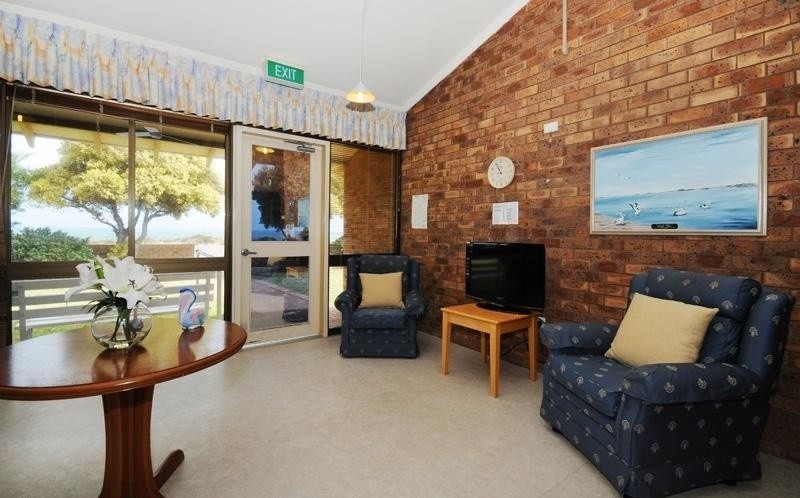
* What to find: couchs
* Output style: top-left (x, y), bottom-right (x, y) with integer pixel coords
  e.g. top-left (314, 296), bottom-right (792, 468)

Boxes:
top-left (539, 268), bottom-right (796, 498)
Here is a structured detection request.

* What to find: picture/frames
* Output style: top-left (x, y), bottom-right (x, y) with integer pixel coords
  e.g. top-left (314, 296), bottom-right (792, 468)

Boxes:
top-left (294, 197), bottom-right (310, 230)
top-left (589, 116), bottom-right (769, 237)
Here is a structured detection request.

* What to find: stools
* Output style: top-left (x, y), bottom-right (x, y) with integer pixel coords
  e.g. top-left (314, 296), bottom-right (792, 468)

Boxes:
top-left (285, 266), bottom-right (308, 279)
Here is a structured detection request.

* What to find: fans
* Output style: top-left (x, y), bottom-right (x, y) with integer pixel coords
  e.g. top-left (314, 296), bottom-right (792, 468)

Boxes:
top-left (115, 126), bottom-right (194, 143)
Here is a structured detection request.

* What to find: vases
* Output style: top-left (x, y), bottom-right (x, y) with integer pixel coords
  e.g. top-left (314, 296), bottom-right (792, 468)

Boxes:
top-left (90, 302), bottom-right (154, 352)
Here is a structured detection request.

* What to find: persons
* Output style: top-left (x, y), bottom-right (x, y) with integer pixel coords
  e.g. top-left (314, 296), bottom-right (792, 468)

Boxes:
top-left (633, 203), bottom-right (641, 216)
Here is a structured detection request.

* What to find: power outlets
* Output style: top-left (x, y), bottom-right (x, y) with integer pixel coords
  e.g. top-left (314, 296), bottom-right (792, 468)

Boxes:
top-left (537, 317), bottom-right (547, 330)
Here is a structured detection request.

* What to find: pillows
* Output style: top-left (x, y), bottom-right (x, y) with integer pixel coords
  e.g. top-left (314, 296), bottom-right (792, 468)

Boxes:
top-left (603, 291), bottom-right (720, 370)
top-left (357, 271), bottom-right (407, 310)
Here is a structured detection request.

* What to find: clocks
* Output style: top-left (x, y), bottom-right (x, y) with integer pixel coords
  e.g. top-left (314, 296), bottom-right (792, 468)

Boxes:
top-left (487, 156), bottom-right (516, 189)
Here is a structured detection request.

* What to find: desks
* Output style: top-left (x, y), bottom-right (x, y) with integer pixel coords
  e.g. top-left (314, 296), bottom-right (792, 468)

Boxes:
top-left (439, 302), bottom-right (539, 399)
top-left (0, 314), bottom-right (247, 498)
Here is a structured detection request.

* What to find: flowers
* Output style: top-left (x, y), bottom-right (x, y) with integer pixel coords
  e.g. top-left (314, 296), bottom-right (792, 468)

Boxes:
top-left (63, 254), bottom-right (169, 344)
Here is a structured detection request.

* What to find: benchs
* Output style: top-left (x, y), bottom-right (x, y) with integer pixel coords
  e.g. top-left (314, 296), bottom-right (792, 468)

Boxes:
top-left (12, 270), bottom-right (223, 342)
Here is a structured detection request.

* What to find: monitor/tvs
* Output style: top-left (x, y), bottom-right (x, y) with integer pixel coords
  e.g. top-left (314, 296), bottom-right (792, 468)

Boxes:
top-left (465, 241), bottom-right (546, 314)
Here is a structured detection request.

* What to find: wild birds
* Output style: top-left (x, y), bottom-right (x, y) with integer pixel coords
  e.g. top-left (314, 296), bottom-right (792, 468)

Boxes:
top-left (701, 200), bottom-right (713, 209)
top-left (629, 202), bottom-right (641, 215)
top-left (615, 209), bottom-right (625, 225)
top-left (674, 207), bottom-right (687, 216)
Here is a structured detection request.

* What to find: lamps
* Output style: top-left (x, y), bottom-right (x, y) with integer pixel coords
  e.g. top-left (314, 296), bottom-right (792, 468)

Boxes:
top-left (255, 146), bottom-right (274, 154)
top-left (342, 1), bottom-right (376, 104)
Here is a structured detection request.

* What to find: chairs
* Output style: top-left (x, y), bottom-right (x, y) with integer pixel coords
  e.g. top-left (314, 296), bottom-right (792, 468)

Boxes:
top-left (334, 254), bottom-right (427, 359)
